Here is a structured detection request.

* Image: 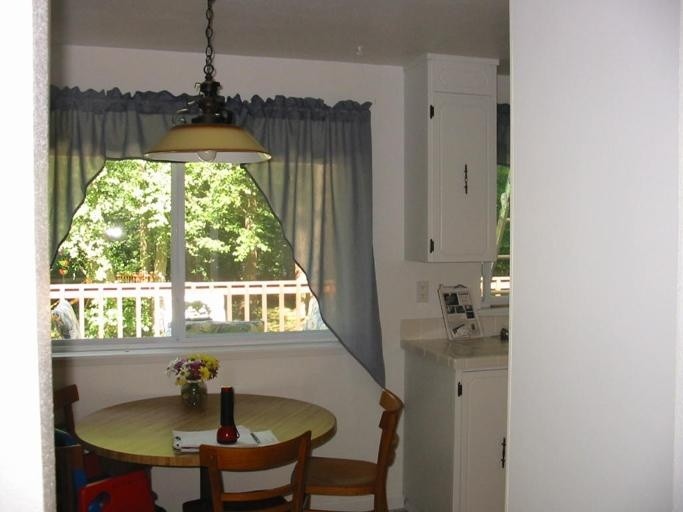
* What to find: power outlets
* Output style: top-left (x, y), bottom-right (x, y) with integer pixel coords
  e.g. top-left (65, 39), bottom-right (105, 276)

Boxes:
top-left (416, 280), bottom-right (428, 302)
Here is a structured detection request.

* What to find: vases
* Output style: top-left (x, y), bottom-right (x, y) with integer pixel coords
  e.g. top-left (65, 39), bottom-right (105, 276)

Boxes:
top-left (182, 382), bottom-right (206, 410)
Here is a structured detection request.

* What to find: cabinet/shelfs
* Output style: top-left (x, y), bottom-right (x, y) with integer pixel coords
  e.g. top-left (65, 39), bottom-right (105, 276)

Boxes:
top-left (400, 52), bottom-right (500, 264)
top-left (401, 347), bottom-right (507, 512)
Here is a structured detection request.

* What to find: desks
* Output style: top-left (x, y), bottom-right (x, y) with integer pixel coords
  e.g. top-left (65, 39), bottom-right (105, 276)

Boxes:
top-left (168, 319), bottom-right (264, 335)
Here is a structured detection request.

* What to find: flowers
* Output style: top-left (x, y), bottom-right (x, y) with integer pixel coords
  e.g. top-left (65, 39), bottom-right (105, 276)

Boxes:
top-left (164, 352), bottom-right (220, 384)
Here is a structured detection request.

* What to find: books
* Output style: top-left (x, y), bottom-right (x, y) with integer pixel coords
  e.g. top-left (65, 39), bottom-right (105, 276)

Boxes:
top-left (171, 424), bottom-right (280, 452)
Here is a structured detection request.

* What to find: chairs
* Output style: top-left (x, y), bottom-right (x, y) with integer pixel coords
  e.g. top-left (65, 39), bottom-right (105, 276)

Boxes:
top-left (293, 295), bottom-right (337, 330)
top-left (179, 432), bottom-right (312, 510)
top-left (52, 300), bottom-right (81, 340)
top-left (54, 384), bottom-right (158, 512)
top-left (289, 389), bottom-right (404, 510)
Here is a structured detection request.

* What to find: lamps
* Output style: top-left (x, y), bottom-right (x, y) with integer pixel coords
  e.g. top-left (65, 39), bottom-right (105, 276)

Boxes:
top-left (142, 0), bottom-right (272, 165)
